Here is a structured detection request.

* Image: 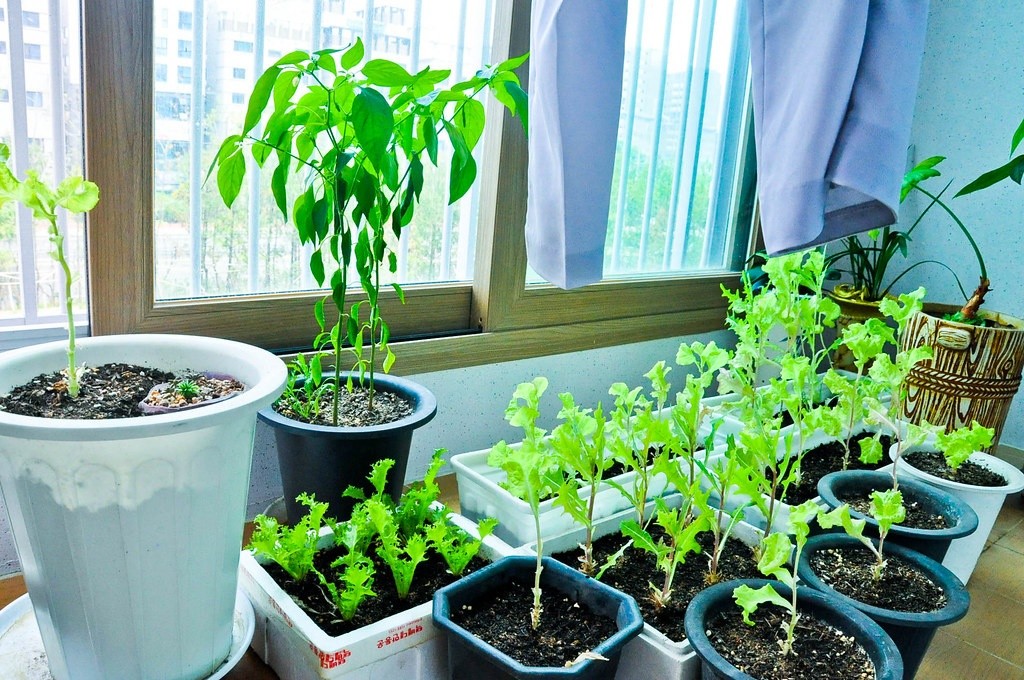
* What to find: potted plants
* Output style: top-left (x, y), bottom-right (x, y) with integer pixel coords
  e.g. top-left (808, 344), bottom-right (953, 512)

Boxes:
top-left (1, 52), bottom-right (1024, 678)
